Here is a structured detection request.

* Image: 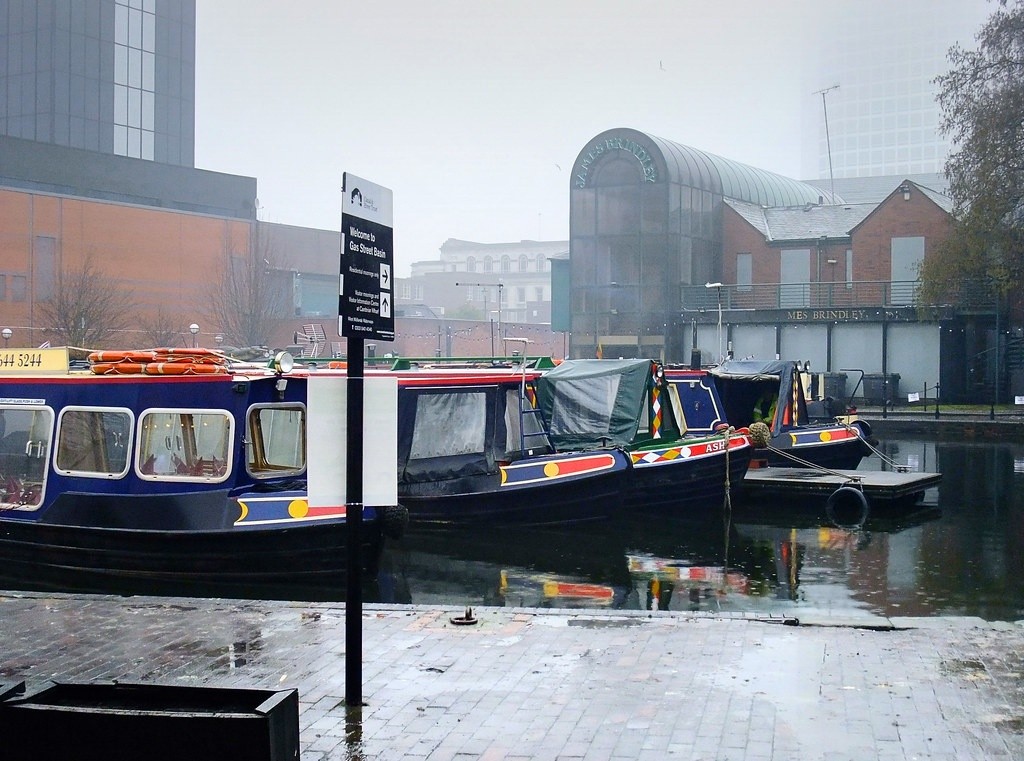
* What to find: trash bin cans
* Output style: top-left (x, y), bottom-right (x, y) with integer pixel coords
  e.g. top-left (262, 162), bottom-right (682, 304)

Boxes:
top-left (816, 372), bottom-right (848, 399)
top-left (862, 372), bottom-right (901, 406)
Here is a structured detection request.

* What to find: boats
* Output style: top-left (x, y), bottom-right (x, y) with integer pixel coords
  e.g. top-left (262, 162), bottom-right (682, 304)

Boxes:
top-left (345, 355), bottom-right (632, 527)
top-left (710, 361), bottom-right (877, 471)
top-left (540, 358), bottom-right (753, 503)
top-left (0, 349), bottom-right (399, 587)
